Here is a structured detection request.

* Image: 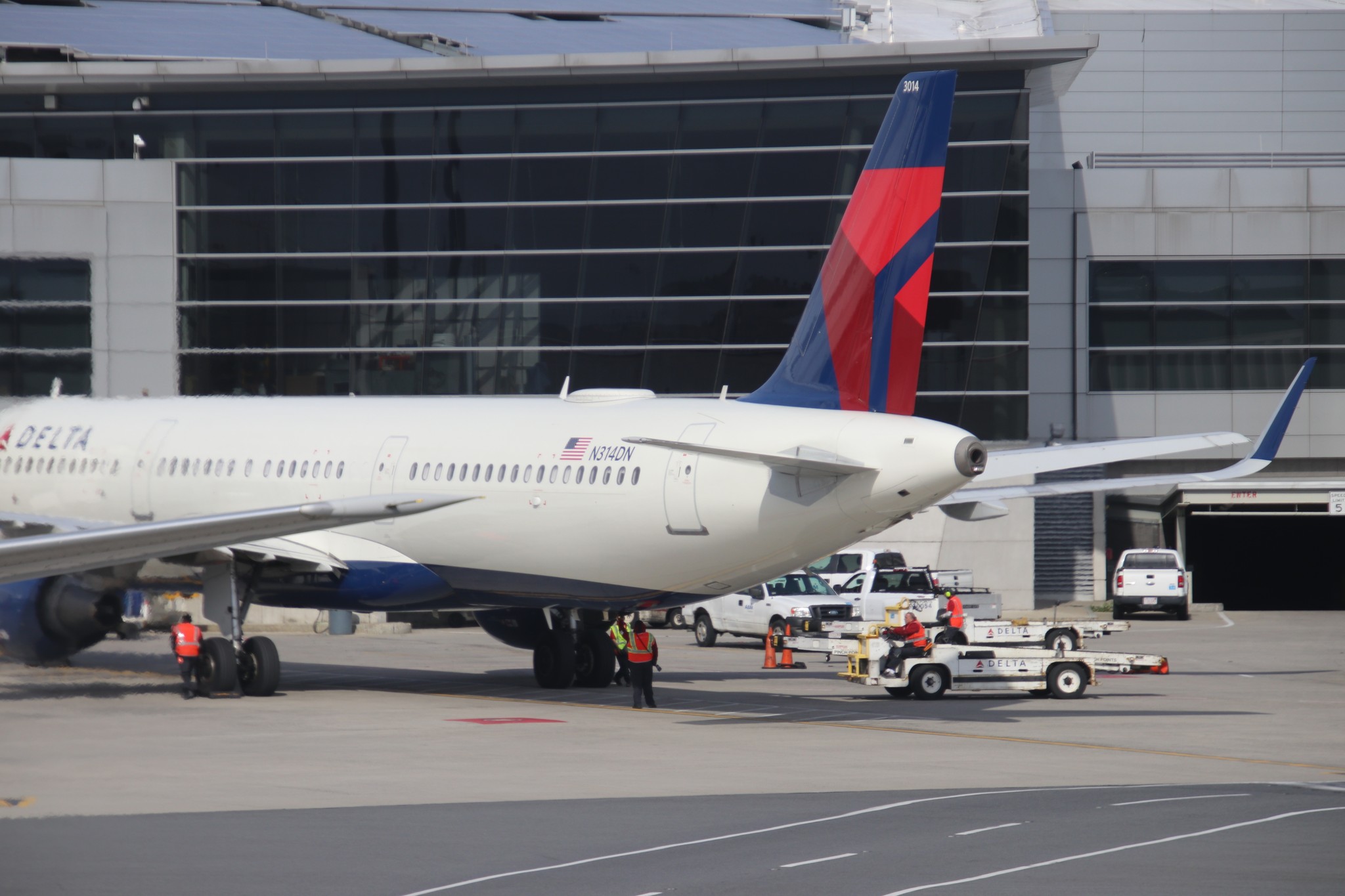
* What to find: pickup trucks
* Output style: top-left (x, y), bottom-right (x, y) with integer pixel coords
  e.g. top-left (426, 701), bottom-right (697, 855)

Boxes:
top-left (1110, 548), bottom-right (1190, 620)
top-left (808, 550), bottom-right (973, 594)
top-left (833, 566), bottom-right (1002, 627)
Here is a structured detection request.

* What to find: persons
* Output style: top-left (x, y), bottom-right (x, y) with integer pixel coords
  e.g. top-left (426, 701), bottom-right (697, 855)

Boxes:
top-left (936, 588), bottom-right (964, 643)
top-left (610, 615), bottom-right (632, 686)
top-left (881, 612), bottom-right (927, 678)
top-left (170, 613), bottom-right (209, 698)
top-left (627, 620), bottom-right (659, 709)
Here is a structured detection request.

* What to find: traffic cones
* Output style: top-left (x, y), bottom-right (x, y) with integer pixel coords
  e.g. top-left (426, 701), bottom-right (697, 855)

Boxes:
top-left (762, 626), bottom-right (780, 670)
top-left (778, 624), bottom-right (799, 667)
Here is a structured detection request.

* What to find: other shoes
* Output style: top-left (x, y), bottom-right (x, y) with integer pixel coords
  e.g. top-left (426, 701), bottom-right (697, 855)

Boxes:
top-left (186, 691), bottom-right (193, 698)
top-left (880, 670), bottom-right (888, 675)
top-left (649, 705), bottom-right (657, 708)
top-left (614, 676), bottom-right (622, 686)
top-left (881, 671), bottom-right (896, 679)
top-left (626, 682), bottom-right (631, 687)
top-left (632, 705), bottom-right (642, 709)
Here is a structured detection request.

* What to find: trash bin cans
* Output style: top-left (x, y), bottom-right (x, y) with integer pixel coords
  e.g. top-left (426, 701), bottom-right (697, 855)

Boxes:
top-left (327, 610), bottom-right (353, 635)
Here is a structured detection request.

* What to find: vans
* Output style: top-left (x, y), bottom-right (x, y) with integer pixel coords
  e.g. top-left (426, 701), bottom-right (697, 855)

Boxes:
top-left (638, 569), bottom-right (857, 651)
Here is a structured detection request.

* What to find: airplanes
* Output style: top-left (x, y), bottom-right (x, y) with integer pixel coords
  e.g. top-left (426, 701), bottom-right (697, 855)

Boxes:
top-left (0, 70), bottom-right (989, 700)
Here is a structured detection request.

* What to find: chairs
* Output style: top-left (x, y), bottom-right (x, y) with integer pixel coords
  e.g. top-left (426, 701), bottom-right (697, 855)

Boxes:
top-left (909, 576), bottom-right (923, 590)
top-left (775, 583), bottom-right (784, 595)
top-left (785, 580), bottom-right (800, 594)
